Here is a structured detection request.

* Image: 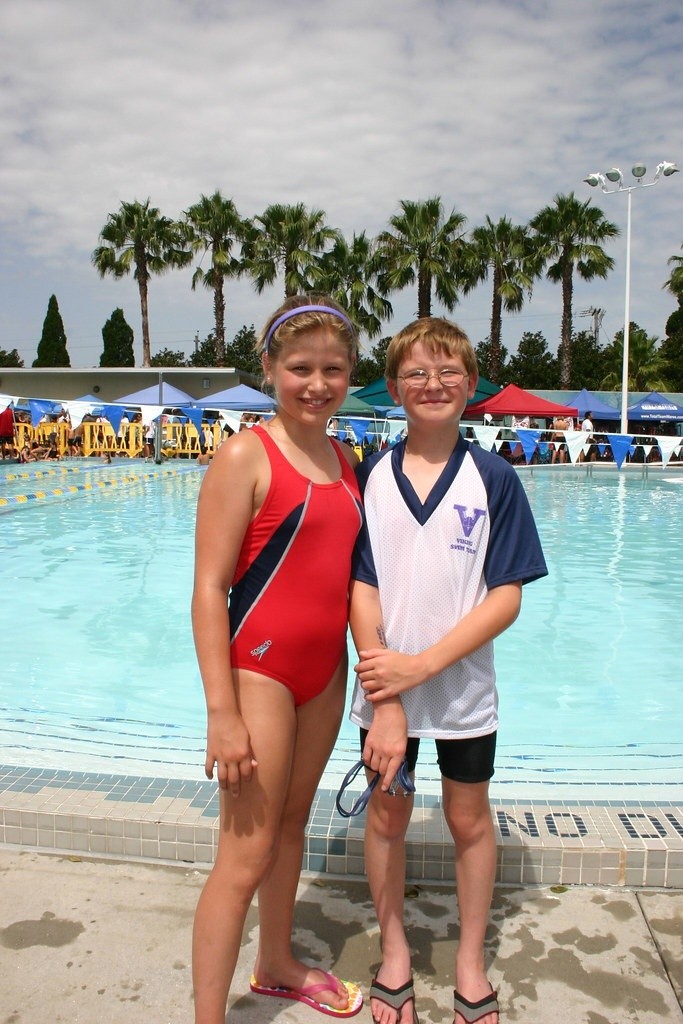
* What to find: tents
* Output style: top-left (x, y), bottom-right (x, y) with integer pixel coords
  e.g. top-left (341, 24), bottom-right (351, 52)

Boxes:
top-left (561, 388), bottom-right (620, 421)
top-left (462, 383), bottom-right (578, 426)
top-left (620, 390), bottom-right (683, 421)
top-left (72, 395), bottom-right (105, 403)
top-left (191, 384), bottom-right (278, 412)
top-left (111, 381), bottom-right (196, 408)
top-left (337, 373), bottom-right (502, 420)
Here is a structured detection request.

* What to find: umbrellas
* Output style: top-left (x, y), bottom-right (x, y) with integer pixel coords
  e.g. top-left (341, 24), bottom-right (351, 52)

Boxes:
top-left (384, 405), bottom-right (407, 419)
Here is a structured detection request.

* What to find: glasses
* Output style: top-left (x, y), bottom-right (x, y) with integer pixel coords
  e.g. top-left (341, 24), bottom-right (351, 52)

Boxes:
top-left (388, 762), bottom-right (416, 798)
top-left (394, 368), bottom-right (471, 389)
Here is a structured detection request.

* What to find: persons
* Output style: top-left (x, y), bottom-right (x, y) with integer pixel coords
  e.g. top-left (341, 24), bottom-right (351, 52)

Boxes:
top-left (0, 404), bottom-right (676, 464)
top-left (117, 414), bottom-right (129, 444)
top-left (328, 415), bottom-right (339, 440)
top-left (101, 453), bottom-right (112, 464)
top-left (143, 420), bottom-right (156, 457)
top-left (350, 317), bottom-right (550, 1024)
top-left (192, 296), bottom-right (365, 1024)
top-left (549, 416), bottom-right (569, 464)
top-left (1, 405), bottom-right (15, 460)
top-left (579, 411), bottom-right (597, 462)
top-left (197, 448), bottom-right (209, 464)
top-left (71, 424), bottom-right (84, 456)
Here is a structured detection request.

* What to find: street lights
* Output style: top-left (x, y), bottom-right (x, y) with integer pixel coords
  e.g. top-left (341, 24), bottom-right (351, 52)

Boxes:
top-left (583, 160), bottom-right (680, 464)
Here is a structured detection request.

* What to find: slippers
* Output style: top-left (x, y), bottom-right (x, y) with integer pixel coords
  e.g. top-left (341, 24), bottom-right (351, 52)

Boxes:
top-left (250, 967), bottom-right (363, 1019)
top-left (369, 967), bottom-right (419, 1024)
top-left (452, 981), bottom-right (499, 1024)
